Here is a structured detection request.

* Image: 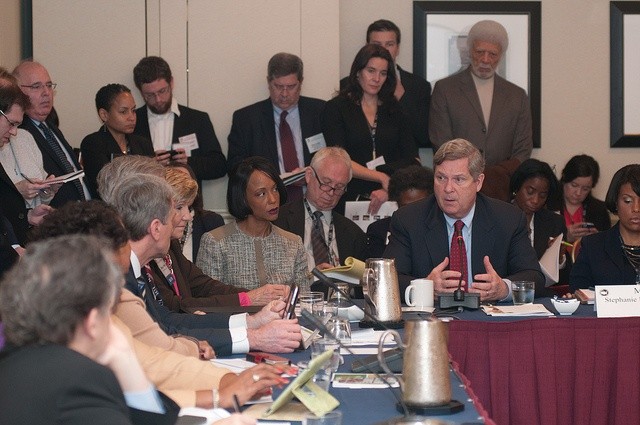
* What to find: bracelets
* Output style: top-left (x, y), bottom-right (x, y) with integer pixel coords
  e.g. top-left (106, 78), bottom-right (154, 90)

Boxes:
top-left (213, 388), bottom-right (218, 408)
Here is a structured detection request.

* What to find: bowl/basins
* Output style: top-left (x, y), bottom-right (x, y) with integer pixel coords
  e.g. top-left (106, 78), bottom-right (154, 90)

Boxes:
top-left (551, 297), bottom-right (582, 316)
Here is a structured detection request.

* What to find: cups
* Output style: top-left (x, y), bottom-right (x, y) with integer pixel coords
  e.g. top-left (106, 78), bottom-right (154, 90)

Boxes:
top-left (327, 283), bottom-right (352, 307)
top-left (324, 319), bottom-right (351, 344)
top-left (303, 411), bottom-right (343, 425)
top-left (404, 279), bottom-right (434, 307)
top-left (511, 281), bottom-right (535, 306)
top-left (311, 340), bottom-right (340, 372)
top-left (300, 292), bottom-right (324, 314)
top-left (312, 302), bottom-right (338, 317)
top-left (298, 361), bottom-right (331, 393)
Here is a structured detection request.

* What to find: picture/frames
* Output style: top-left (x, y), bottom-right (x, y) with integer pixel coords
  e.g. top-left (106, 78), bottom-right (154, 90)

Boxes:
top-left (412, 0), bottom-right (542, 149)
top-left (609, 0), bottom-right (640, 148)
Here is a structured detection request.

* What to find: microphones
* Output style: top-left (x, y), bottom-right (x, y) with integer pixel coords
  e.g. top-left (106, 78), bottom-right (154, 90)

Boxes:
top-left (300, 309), bottom-right (452, 424)
top-left (310, 268), bottom-right (399, 331)
top-left (439, 220), bottom-right (481, 309)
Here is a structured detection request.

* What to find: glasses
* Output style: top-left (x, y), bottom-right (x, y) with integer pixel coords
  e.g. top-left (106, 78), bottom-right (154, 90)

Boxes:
top-left (310, 164), bottom-right (349, 196)
top-left (20, 83), bottom-right (58, 91)
top-left (0, 109), bottom-right (22, 129)
top-left (145, 83), bottom-right (170, 100)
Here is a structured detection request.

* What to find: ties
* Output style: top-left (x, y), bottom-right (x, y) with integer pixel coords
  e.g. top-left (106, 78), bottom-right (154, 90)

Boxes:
top-left (279, 111), bottom-right (300, 173)
top-left (310, 210), bottom-right (335, 270)
top-left (143, 264), bottom-right (166, 310)
top-left (449, 221), bottom-right (469, 294)
top-left (39, 123), bottom-right (86, 205)
top-left (135, 271), bottom-right (147, 301)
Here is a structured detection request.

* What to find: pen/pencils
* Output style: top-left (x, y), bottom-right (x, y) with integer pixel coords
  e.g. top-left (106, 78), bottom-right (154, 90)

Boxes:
top-left (231, 393), bottom-right (241, 414)
top-left (19, 172), bottom-right (47, 195)
top-left (548, 236), bottom-right (573, 247)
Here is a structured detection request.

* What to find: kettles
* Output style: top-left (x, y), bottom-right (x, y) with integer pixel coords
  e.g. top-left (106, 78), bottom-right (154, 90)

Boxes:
top-left (377, 318), bottom-right (465, 416)
top-left (359, 258), bottom-right (405, 328)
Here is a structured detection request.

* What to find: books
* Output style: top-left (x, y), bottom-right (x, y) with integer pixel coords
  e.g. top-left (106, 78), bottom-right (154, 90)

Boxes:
top-left (479, 303), bottom-right (554, 318)
top-left (331, 372), bottom-right (404, 389)
top-left (538, 233), bottom-right (563, 291)
top-left (310, 256), bottom-right (366, 286)
top-left (42, 171), bottom-right (87, 183)
top-left (277, 166), bottom-right (308, 187)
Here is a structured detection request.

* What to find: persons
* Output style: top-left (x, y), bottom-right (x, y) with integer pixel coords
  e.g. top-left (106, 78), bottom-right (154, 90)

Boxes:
top-left (145, 164), bottom-right (291, 318)
top-left (22, 206), bottom-right (298, 410)
top-left (1, 238), bottom-right (180, 425)
top-left (380, 138), bottom-right (545, 305)
top-left (94, 153), bottom-right (161, 205)
top-left (1, 83), bottom-right (55, 277)
top-left (128, 55), bottom-right (228, 212)
top-left (226, 52), bottom-right (327, 224)
top-left (327, 45), bottom-right (420, 215)
top-left (0, 67), bottom-right (87, 212)
top-left (572, 163), bottom-right (639, 297)
top-left (557, 154), bottom-right (611, 262)
top-left (429, 20), bottom-right (533, 203)
top-left (108, 173), bottom-right (302, 357)
top-left (12, 60), bottom-right (95, 204)
top-left (508, 159), bottom-right (567, 288)
top-left (273, 147), bottom-right (368, 300)
top-left (194, 158), bottom-right (314, 303)
top-left (364, 164), bottom-right (435, 265)
top-left (79, 82), bottom-right (140, 203)
top-left (340, 19), bottom-right (431, 167)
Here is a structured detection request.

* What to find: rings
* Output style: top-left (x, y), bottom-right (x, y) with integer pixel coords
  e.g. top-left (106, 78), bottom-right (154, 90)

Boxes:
top-left (251, 373), bottom-right (259, 382)
top-left (486, 291), bottom-right (489, 298)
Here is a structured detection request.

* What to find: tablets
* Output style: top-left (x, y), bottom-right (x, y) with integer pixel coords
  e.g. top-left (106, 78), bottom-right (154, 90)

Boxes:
top-left (255, 348), bottom-right (340, 420)
top-left (281, 283), bottom-right (299, 319)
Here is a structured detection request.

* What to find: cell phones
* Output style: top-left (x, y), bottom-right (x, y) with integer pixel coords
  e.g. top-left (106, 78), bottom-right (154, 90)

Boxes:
top-left (160, 150), bottom-right (184, 162)
top-left (582, 222), bottom-right (593, 228)
